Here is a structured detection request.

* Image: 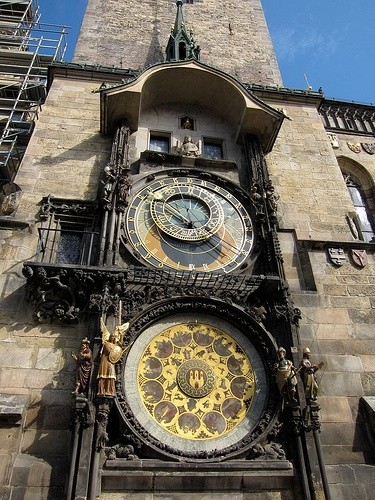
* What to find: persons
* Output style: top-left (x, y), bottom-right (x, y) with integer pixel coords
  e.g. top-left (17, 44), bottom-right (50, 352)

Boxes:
top-left (71, 337), bottom-right (92, 396)
top-left (101, 166), bottom-right (115, 201)
top-left (252, 440), bottom-right (288, 459)
top-left (96, 317), bottom-right (129, 398)
top-left (274, 346), bottom-right (298, 405)
top-left (252, 183), bottom-right (265, 216)
top-left (118, 168), bottom-right (131, 204)
top-left (300, 348), bottom-right (320, 405)
top-left (266, 186), bottom-right (280, 218)
top-left (105, 442), bottom-right (134, 460)
top-left (180, 135), bottom-right (198, 158)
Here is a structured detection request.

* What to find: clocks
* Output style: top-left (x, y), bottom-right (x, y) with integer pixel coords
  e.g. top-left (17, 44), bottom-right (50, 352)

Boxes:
top-left (118, 165), bottom-right (264, 283)
